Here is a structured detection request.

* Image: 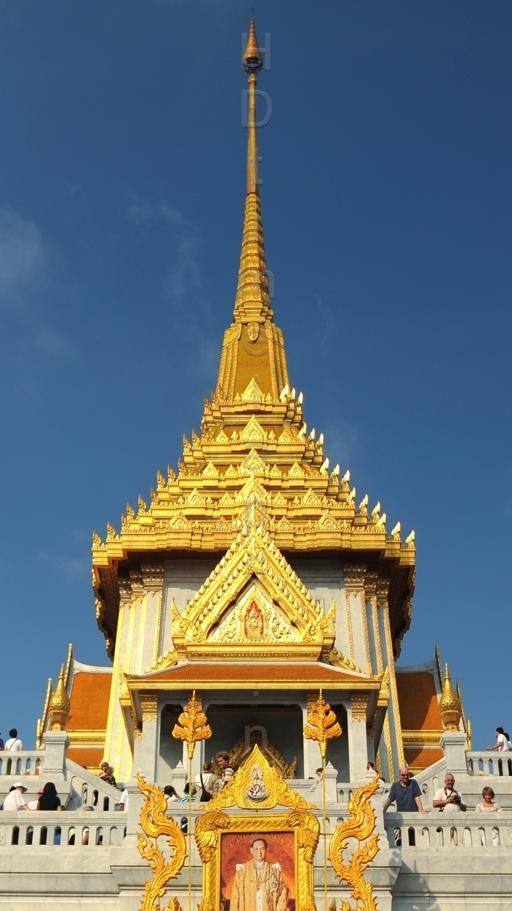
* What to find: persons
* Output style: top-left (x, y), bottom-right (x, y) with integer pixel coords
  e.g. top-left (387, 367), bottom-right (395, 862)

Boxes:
top-left (99, 761), bottom-right (116, 786)
top-left (163, 751), bottom-right (235, 802)
top-left (367, 761), bottom-right (374, 770)
top-left (0, 728), bottom-right (23, 751)
top-left (82, 805), bottom-right (100, 845)
top-left (3, 782), bottom-right (30, 811)
top-left (475, 786), bottom-right (503, 847)
top-left (27, 782), bottom-right (62, 811)
top-left (229, 838), bottom-right (289, 911)
top-left (433, 772), bottom-right (467, 847)
top-left (487, 727), bottom-right (512, 776)
top-left (316, 768), bottom-right (323, 777)
top-left (383, 767), bottom-right (424, 845)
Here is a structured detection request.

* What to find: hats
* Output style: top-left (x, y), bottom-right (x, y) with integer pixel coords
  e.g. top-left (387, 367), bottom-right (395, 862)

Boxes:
top-left (13, 782), bottom-right (28, 793)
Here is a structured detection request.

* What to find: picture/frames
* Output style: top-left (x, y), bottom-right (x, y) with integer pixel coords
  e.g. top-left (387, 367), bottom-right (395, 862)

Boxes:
top-left (193, 808), bottom-right (321, 911)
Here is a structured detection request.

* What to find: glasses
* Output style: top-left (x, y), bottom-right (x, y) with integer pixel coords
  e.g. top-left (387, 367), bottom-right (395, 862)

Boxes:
top-left (400, 774), bottom-right (409, 777)
